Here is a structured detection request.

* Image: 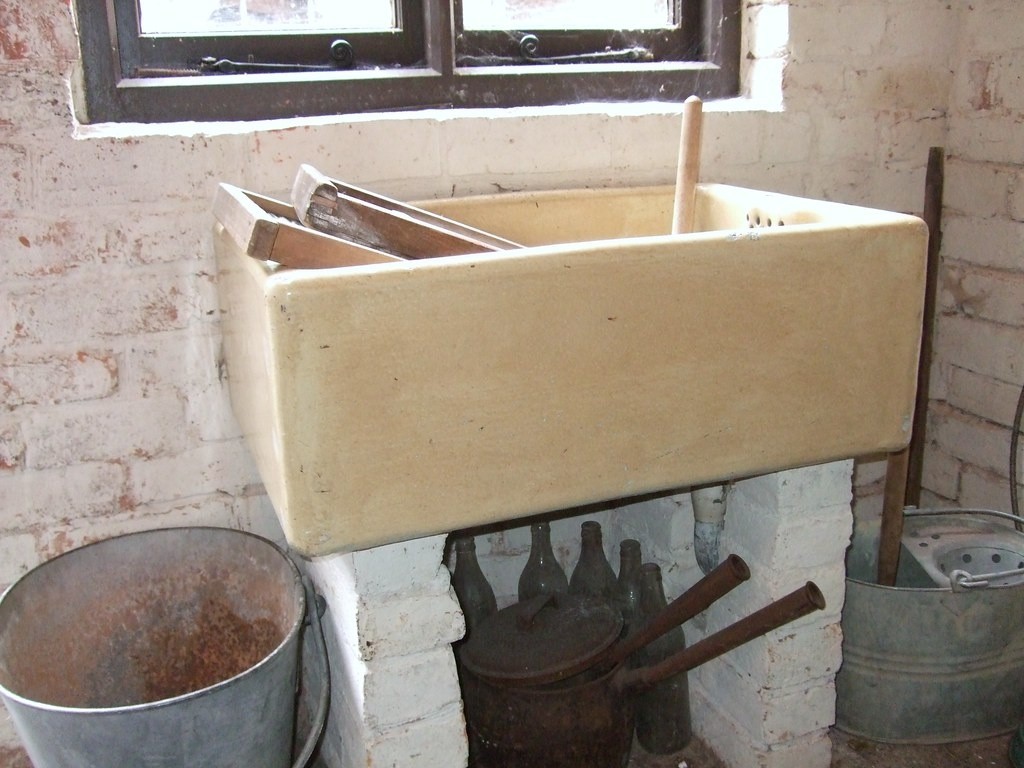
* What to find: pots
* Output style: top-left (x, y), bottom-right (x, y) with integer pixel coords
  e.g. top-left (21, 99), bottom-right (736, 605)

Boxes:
top-left (455, 554), bottom-right (827, 768)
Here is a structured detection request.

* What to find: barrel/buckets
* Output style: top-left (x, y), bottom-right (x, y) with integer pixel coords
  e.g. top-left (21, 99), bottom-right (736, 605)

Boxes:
top-left (830, 506), bottom-right (1024, 745)
top-left (0, 525), bottom-right (332, 768)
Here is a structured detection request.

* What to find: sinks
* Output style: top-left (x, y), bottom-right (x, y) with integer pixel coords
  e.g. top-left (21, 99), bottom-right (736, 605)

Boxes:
top-left (210, 183), bottom-right (930, 558)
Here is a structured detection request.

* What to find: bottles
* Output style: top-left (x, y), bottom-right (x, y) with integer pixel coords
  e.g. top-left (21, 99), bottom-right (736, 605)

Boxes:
top-left (628, 563), bottom-right (692, 756)
top-left (451, 536), bottom-right (497, 651)
top-left (569, 520), bottom-right (618, 608)
top-left (517, 519), bottom-right (569, 601)
top-left (616, 538), bottom-right (643, 637)
